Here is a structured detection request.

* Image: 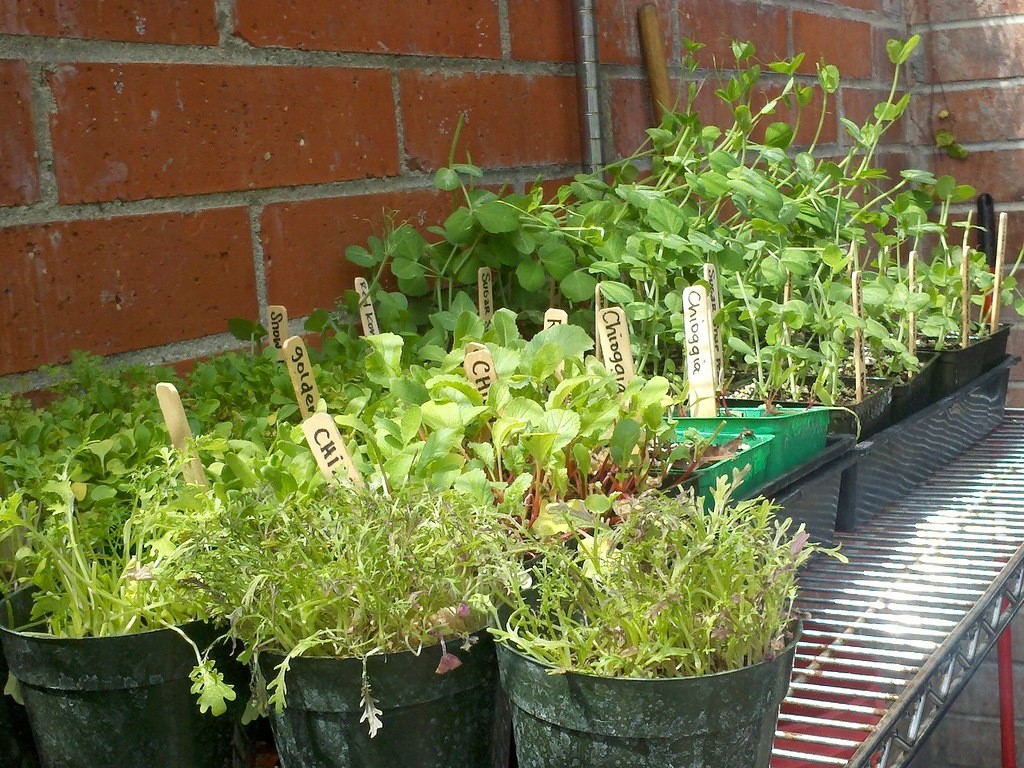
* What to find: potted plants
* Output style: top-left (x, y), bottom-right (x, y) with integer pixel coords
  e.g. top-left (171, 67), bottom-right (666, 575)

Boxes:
top-left (0, 38), bottom-right (1024, 768)
